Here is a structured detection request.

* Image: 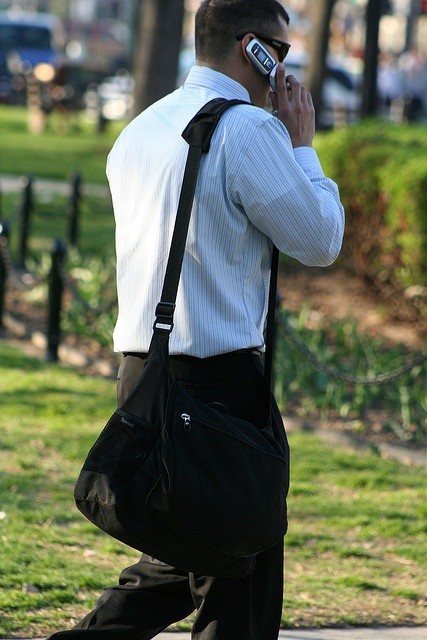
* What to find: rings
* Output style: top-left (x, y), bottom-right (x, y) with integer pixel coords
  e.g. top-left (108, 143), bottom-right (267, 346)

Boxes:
top-left (273, 110), bottom-right (278, 116)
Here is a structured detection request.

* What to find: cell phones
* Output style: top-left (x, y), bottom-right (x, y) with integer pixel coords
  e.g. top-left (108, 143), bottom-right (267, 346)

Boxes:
top-left (244, 38), bottom-right (291, 92)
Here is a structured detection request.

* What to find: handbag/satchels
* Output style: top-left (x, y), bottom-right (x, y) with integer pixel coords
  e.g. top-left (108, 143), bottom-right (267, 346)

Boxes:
top-left (72, 96), bottom-right (290, 582)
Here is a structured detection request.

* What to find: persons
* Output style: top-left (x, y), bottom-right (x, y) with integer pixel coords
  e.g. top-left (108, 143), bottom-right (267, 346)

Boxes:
top-left (42, 0), bottom-right (348, 639)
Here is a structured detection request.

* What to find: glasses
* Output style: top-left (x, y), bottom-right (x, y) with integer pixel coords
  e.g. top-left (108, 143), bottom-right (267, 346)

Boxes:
top-left (235, 29), bottom-right (291, 62)
